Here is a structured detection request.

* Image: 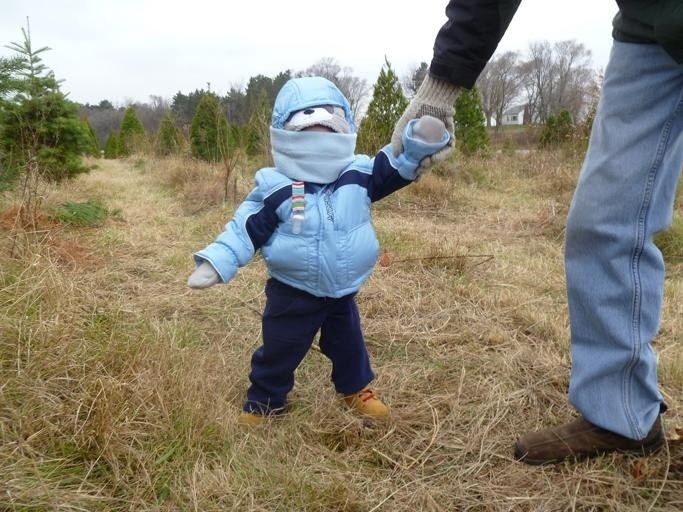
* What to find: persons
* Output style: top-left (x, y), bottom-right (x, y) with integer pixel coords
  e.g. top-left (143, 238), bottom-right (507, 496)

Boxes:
top-left (389, 0), bottom-right (681, 467)
top-left (184, 74), bottom-right (452, 432)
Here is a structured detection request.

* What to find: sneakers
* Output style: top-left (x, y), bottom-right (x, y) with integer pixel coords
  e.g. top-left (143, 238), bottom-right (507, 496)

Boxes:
top-left (236, 410), bottom-right (270, 430)
top-left (341, 382), bottom-right (389, 420)
top-left (513, 412), bottom-right (665, 467)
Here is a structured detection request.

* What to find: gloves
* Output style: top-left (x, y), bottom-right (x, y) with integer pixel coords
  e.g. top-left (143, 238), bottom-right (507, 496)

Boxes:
top-left (410, 114), bottom-right (446, 144)
top-left (389, 71), bottom-right (463, 183)
top-left (186, 259), bottom-right (219, 290)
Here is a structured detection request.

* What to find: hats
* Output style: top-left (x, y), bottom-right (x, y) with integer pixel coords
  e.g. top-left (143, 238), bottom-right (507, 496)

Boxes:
top-left (281, 102), bottom-right (351, 136)
